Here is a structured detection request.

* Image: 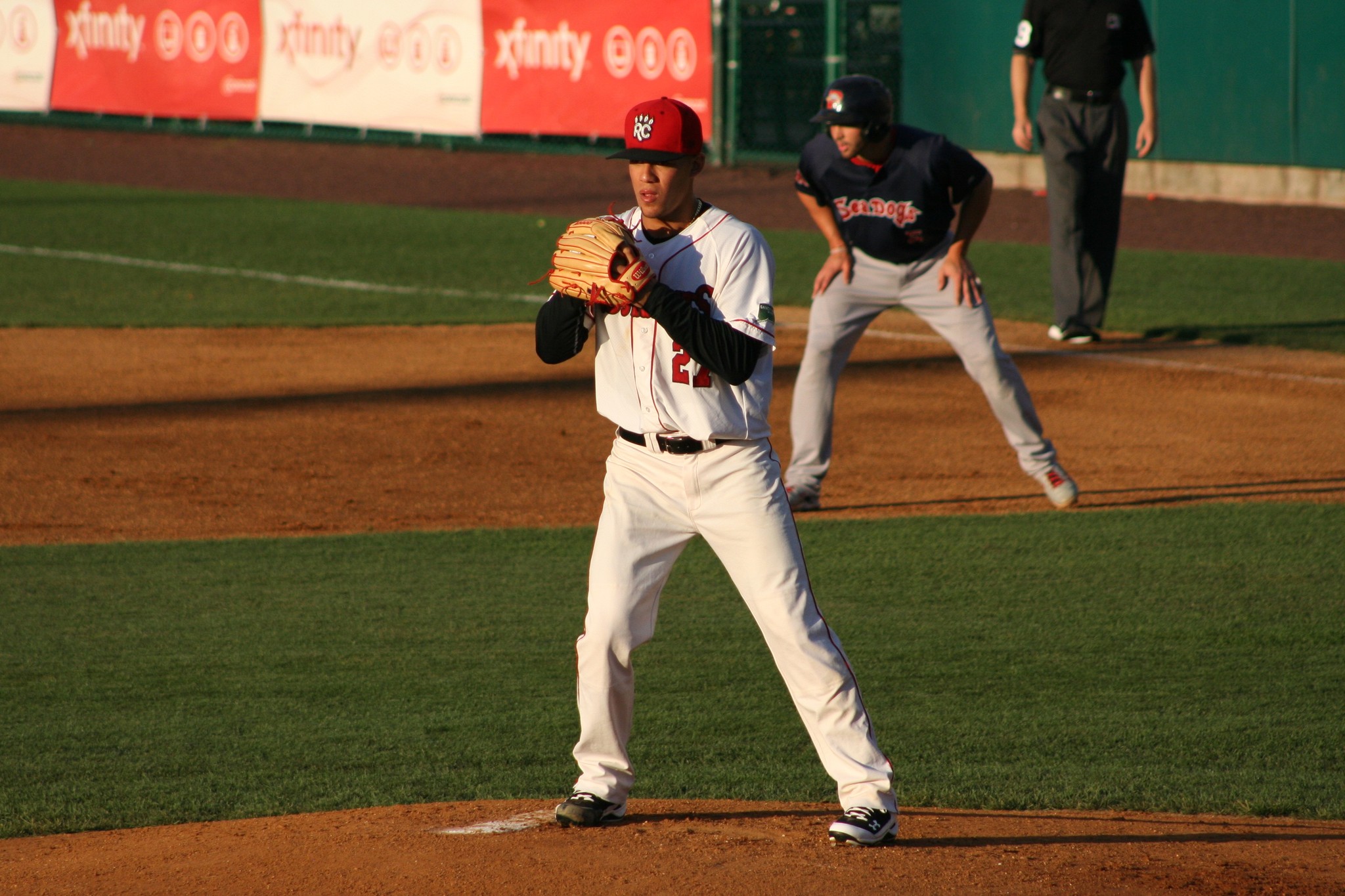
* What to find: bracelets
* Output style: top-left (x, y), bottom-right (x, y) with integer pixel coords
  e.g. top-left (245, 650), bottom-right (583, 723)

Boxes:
top-left (831, 247), bottom-right (846, 253)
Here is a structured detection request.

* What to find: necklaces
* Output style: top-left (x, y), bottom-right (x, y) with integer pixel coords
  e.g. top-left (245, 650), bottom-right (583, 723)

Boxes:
top-left (691, 200), bottom-right (702, 223)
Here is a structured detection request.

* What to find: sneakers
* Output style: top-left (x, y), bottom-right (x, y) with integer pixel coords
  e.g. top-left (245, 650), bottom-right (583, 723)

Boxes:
top-left (1032, 461), bottom-right (1078, 508)
top-left (825, 806), bottom-right (902, 845)
top-left (780, 484), bottom-right (826, 512)
top-left (553, 790), bottom-right (628, 827)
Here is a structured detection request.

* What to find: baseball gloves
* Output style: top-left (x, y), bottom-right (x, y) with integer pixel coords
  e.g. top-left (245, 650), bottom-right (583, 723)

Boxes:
top-left (549, 215), bottom-right (657, 318)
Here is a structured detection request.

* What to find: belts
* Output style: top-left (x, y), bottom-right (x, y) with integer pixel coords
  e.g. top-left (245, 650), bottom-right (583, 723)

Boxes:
top-left (617, 427), bottom-right (733, 454)
top-left (1050, 85), bottom-right (1123, 105)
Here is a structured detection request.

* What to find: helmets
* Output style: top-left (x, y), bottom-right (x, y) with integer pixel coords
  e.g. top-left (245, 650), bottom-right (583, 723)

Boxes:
top-left (808, 74), bottom-right (896, 128)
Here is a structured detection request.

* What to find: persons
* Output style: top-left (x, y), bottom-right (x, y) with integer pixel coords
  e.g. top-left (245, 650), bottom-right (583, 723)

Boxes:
top-left (1010, 0), bottom-right (1155, 345)
top-left (536, 97), bottom-right (897, 843)
top-left (781, 74), bottom-right (1080, 510)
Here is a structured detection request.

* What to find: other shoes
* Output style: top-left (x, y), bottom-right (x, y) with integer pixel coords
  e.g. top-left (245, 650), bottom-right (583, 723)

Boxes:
top-left (1047, 322), bottom-right (1097, 346)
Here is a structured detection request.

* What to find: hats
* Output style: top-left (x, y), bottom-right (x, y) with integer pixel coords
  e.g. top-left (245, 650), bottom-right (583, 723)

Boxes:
top-left (604, 95), bottom-right (705, 163)
